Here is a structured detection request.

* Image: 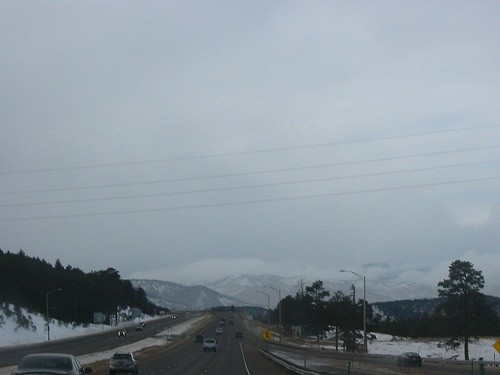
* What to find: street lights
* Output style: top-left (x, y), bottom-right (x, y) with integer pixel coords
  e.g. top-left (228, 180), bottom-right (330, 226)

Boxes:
top-left (47, 287), bottom-right (62, 341)
top-left (340, 270), bottom-right (367, 352)
top-left (255, 290), bottom-right (270, 328)
top-left (264, 285), bottom-right (281, 343)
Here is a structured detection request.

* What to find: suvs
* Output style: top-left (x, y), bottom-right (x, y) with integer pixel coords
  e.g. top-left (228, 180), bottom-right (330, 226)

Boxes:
top-left (203, 338), bottom-right (217, 351)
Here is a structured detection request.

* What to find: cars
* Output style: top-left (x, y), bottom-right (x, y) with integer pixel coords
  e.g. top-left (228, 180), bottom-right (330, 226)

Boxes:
top-left (136, 322), bottom-right (145, 331)
top-left (218, 318), bottom-right (233, 326)
top-left (10, 353), bottom-right (92, 375)
top-left (235, 331), bottom-right (242, 338)
top-left (216, 327), bottom-right (223, 334)
top-left (399, 352), bottom-right (422, 367)
top-left (118, 329), bottom-right (126, 336)
top-left (172, 316), bottom-right (176, 319)
top-left (108, 352), bottom-right (138, 375)
top-left (195, 335), bottom-right (204, 342)
